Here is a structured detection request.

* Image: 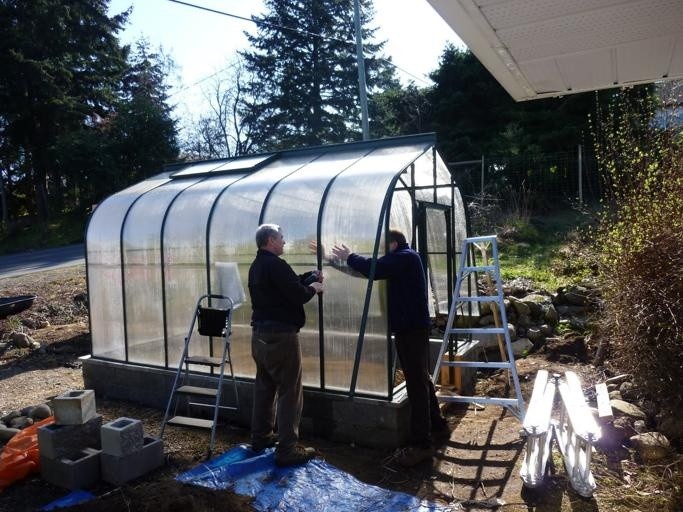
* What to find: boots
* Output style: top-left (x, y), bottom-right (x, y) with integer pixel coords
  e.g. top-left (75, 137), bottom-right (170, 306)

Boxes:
top-left (250, 430), bottom-right (278, 453)
top-left (275, 443), bottom-right (317, 469)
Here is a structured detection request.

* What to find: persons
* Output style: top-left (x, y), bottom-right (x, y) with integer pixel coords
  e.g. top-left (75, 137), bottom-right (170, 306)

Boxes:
top-left (246, 224), bottom-right (325, 466)
top-left (309, 229), bottom-right (450, 472)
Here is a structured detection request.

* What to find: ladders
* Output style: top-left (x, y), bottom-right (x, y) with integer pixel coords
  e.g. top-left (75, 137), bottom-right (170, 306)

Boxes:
top-left (432, 235), bottom-right (526, 422)
top-left (518, 369), bottom-right (603, 497)
top-left (157, 294), bottom-right (240, 460)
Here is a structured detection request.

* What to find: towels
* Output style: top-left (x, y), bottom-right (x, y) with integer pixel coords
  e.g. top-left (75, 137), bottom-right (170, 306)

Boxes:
top-left (215, 263), bottom-right (247, 310)
